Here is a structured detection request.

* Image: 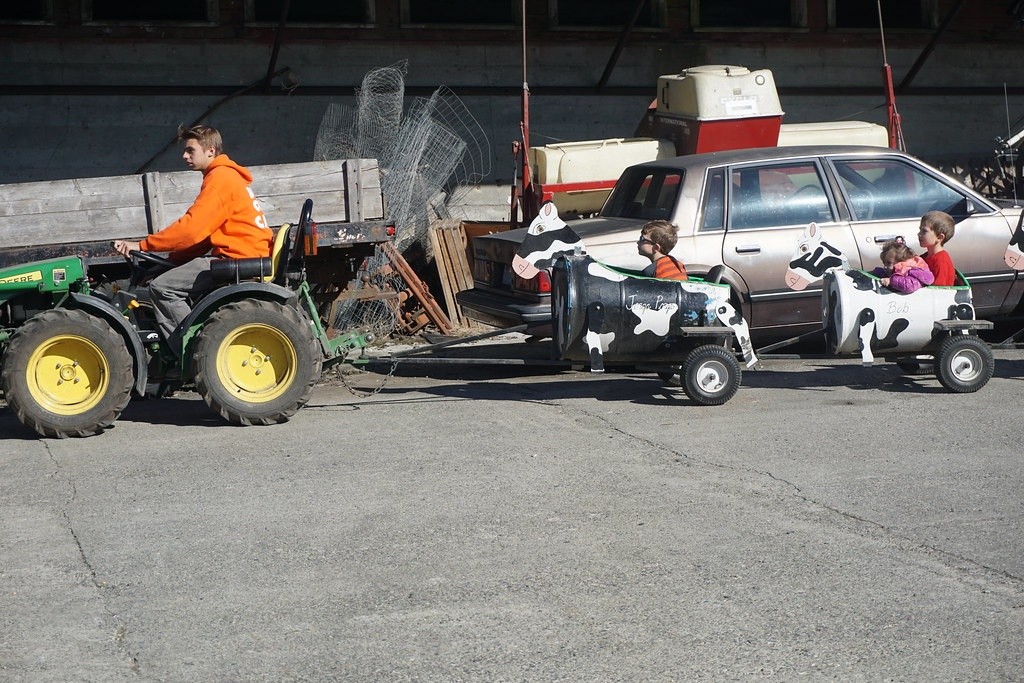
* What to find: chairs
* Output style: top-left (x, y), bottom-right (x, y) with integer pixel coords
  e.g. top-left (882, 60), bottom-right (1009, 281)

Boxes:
top-left (216, 223), bottom-right (294, 283)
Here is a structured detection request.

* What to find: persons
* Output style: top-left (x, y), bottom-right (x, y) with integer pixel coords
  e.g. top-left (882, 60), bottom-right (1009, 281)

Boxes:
top-left (636, 219), bottom-right (689, 281)
top-left (868, 242), bottom-right (934, 295)
top-left (114, 125), bottom-right (275, 383)
top-left (917, 211), bottom-right (955, 287)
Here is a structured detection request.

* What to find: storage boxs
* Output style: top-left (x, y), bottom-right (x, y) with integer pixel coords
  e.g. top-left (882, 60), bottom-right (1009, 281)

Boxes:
top-left (655, 65), bottom-right (785, 155)
top-left (527, 135), bottom-right (676, 213)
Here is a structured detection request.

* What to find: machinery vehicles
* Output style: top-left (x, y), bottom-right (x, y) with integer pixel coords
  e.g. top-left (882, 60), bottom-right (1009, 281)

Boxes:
top-left (0, 198), bottom-right (375, 440)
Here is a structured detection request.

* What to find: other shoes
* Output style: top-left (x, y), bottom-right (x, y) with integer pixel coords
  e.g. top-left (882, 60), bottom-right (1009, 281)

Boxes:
top-left (165, 365), bottom-right (182, 379)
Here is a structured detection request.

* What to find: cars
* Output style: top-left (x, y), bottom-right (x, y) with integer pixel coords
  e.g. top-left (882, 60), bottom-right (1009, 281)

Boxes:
top-left (455, 145), bottom-right (1023, 338)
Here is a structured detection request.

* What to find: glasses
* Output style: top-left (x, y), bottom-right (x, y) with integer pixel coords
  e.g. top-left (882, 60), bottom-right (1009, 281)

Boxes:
top-left (639, 236), bottom-right (663, 251)
